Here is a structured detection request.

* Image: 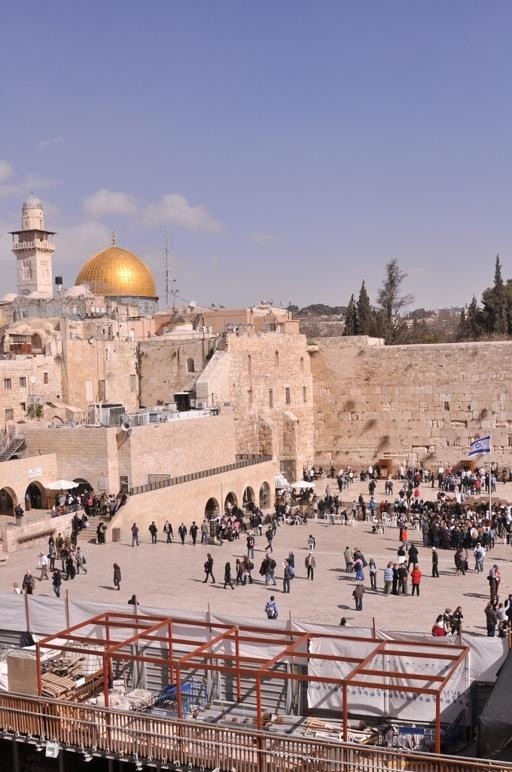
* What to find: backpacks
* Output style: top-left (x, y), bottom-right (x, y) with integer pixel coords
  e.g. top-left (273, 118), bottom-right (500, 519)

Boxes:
top-left (288, 565), bottom-right (294, 576)
top-left (476, 551), bottom-right (482, 560)
top-left (355, 562), bottom-right (361, 572)
top-left (249, 540), bottom-right (254, 547)
top-left (267, 607), bottom-right (275, 617)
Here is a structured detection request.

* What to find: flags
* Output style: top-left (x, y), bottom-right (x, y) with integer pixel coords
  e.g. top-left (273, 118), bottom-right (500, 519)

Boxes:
top-left (468, 435), bottom-right (490, 457)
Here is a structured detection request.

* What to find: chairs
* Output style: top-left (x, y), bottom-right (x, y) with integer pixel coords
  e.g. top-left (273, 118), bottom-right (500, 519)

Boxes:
top-left (282, 512), bottom-right (420, 536)
